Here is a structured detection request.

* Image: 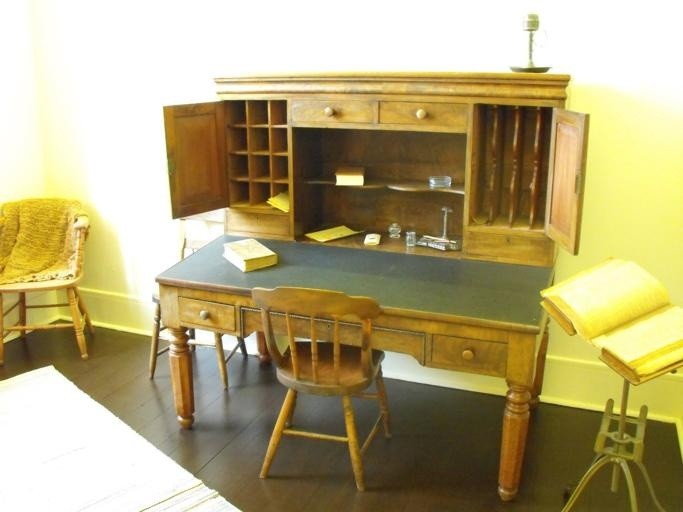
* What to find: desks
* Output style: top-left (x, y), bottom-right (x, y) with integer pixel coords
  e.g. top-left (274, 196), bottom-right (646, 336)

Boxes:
top-left (155, 231), bottom-right (556, 502)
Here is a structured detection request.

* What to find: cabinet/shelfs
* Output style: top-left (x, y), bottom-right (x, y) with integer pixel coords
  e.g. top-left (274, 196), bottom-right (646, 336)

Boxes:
top-left (162, 72), bottom-right (591, 269)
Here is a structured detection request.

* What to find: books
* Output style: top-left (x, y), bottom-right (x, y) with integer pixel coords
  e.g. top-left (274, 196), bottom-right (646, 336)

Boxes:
top-left (538, 253), bottom-right (683, 385)
top-left (333, 164), bottom-right (367, 187)
top-left (223, 238), bottom-right (278, 273)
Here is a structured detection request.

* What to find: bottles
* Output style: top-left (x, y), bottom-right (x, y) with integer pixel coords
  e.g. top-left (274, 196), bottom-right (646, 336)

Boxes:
top-left (406, 231), bottom-right (416, 248)
top-left (388, 223), bottom-right (401, 240)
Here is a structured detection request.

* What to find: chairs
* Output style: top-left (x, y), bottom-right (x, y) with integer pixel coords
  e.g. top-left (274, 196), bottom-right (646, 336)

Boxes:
top-left (0, 195), bottom-right (97, 362)
top-left (147, 235), bottom-right (248, 391)
top-left (251, 285), bottom-right (393, 492)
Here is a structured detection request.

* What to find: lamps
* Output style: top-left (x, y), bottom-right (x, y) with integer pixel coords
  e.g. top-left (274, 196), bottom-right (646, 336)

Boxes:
top-left (510, 13), bottom-right (551, 73)
